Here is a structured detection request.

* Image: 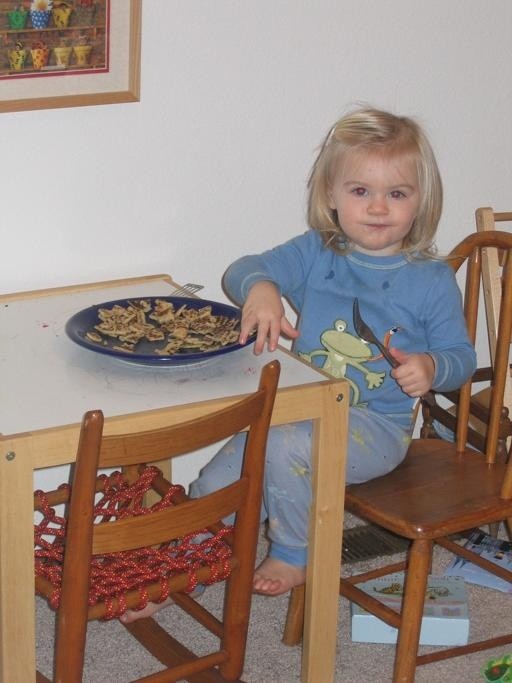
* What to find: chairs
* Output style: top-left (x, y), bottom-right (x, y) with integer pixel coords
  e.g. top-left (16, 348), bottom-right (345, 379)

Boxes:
top-left (33, 360), bottom-right (281, 683)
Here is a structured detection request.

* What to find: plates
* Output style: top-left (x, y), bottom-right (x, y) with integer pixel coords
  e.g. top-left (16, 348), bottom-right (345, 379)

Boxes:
top-left (65, 295), bottom-right (259, 369)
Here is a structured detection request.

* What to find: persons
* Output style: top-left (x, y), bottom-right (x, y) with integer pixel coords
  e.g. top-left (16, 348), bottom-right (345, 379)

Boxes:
top-left (117, 108), bottom-right (479, 626)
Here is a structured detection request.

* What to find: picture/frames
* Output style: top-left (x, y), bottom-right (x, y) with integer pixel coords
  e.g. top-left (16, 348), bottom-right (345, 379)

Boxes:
top-left (0, 0), bottom-right (142, 113)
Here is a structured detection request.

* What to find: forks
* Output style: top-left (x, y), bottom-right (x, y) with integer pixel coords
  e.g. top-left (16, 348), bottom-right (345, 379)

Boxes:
top-left (352, 297), bottom-right (437, 409)
